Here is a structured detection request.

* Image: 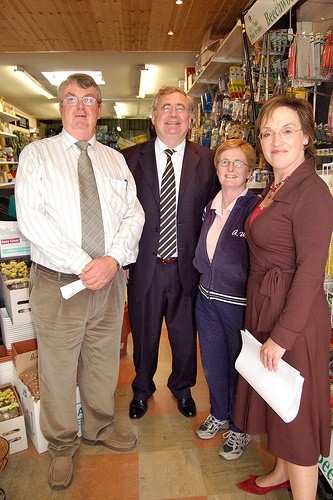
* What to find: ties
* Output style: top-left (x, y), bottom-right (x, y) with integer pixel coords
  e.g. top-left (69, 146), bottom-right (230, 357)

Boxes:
top-left (157, 148), bottom-right (178, 259)
top-left (75, 140), bottom-right (105, 259)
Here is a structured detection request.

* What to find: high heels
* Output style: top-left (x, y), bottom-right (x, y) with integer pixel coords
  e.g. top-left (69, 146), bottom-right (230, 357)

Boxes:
top-left (234, 473), bottom-right (292, 495)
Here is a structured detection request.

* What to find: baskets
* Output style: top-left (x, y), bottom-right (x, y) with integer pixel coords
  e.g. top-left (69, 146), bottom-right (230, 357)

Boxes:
top-left (0, 436), bottom-right (10, 471)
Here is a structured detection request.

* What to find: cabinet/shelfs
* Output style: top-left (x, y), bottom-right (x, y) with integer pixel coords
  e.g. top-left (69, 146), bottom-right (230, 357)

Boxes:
top-left (0, 110), bottom-right (19, 189)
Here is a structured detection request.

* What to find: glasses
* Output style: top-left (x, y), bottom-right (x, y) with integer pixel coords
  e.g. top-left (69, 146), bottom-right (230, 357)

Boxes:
top-left (257, 126), bottom-right (303, 141)
top-left (156, 106), bottom-right (189, 113)
top-left (217, 159), bottom-right (249, 168)
top-left (61, 96), bottom-right (97, 106)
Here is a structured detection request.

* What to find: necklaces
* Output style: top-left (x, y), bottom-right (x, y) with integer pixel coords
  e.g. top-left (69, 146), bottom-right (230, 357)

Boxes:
top-left (268, 176), bottom-right (290, 199)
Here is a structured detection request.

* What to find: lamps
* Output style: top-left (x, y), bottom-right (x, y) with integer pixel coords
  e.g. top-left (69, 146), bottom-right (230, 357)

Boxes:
top-left (12, 65), bottom-right (57, 100)
top-left (136, 64), bottom-right (148, 98)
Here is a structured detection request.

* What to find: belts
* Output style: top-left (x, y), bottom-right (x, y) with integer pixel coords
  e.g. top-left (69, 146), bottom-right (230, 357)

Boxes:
top-left (156, 257), bottom-right (178, 264)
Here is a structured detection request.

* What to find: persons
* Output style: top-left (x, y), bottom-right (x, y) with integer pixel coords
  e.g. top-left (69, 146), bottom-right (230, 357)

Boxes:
top-left (14, 74), bottom-right (137, 489)
top-left (233, 96), bottom-right (333, 500)
top-left (118, 86), bottom-right (222, 419)
top-left (196, 141), bottom-right (261, 459)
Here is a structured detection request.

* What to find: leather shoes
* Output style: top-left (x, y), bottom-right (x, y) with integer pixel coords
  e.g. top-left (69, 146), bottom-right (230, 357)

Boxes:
top-left (47, 455), bottom-right (75, 490)
top-left (129, 396), bottom-right (148, 419)
top-left (81, 423), bottom-right (137, 452)
top-left (165, 383), bottom-right (196, 417)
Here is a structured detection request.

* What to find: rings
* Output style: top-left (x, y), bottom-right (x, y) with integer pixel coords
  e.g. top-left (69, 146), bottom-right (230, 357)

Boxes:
top-left (267, 360), bottom-right (272, 361)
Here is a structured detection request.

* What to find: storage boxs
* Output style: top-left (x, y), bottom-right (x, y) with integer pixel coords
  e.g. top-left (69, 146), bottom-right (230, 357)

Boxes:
top-left (184, 24), bottom-right (227, 91)
top-left (0, 221), bottom-right (84, 454)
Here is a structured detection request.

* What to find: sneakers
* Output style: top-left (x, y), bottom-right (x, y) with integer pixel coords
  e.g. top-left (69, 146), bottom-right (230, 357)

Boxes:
top-left (218, 429), bottom-right (253, 461)
top-left (195, 413), bottom-right (230, 440)
